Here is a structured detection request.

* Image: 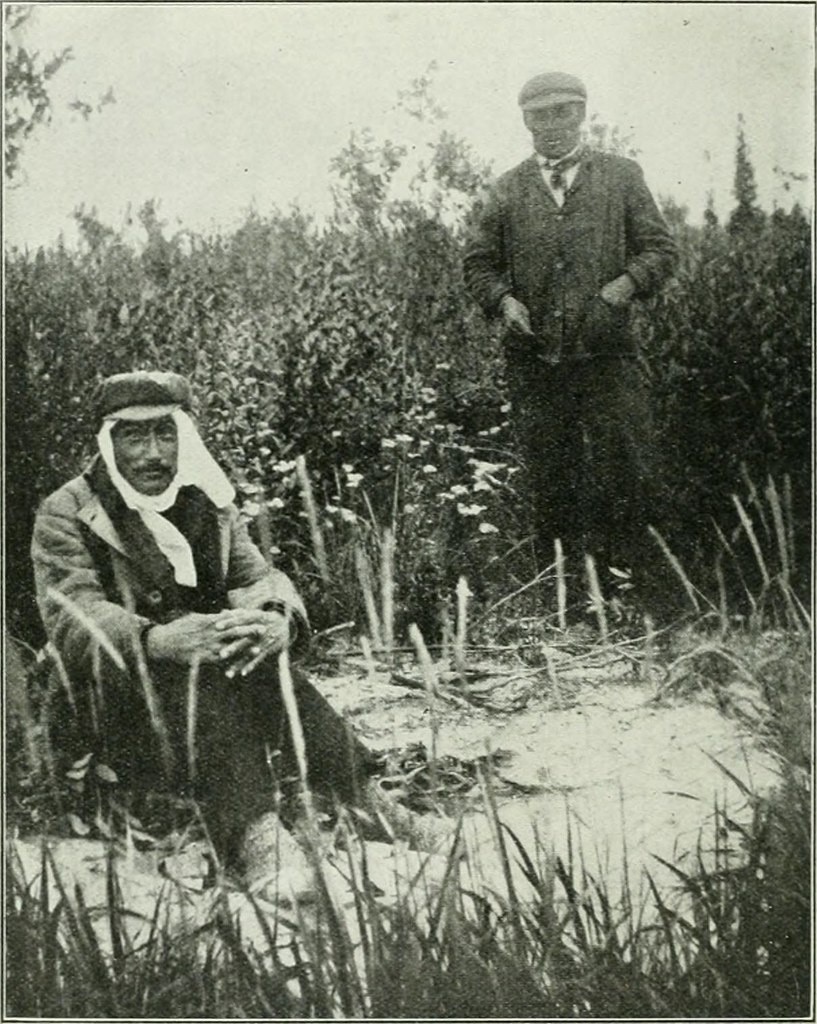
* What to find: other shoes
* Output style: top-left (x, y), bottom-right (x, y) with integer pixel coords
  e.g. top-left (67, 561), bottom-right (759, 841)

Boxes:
top-left (326, 777), bottom-right (478, 856)
top-left (230, 811), bottom-right (318, 906)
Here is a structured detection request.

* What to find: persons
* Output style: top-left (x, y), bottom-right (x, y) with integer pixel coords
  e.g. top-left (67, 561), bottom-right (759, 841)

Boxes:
top-left (31, 370), bottom-right (437, 904)
top-left (462, 70), bottom-right (679, 604)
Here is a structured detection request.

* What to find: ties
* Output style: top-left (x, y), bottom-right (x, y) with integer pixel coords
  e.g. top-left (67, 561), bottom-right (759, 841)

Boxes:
top-left (543, 159), bottom-right (577, 189)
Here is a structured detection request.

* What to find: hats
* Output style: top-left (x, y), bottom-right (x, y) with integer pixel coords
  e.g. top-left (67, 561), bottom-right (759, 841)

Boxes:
top-left (518, 71), bottom-right (587, 109)
top-left (92, 371), bottom-right (192, 422)
